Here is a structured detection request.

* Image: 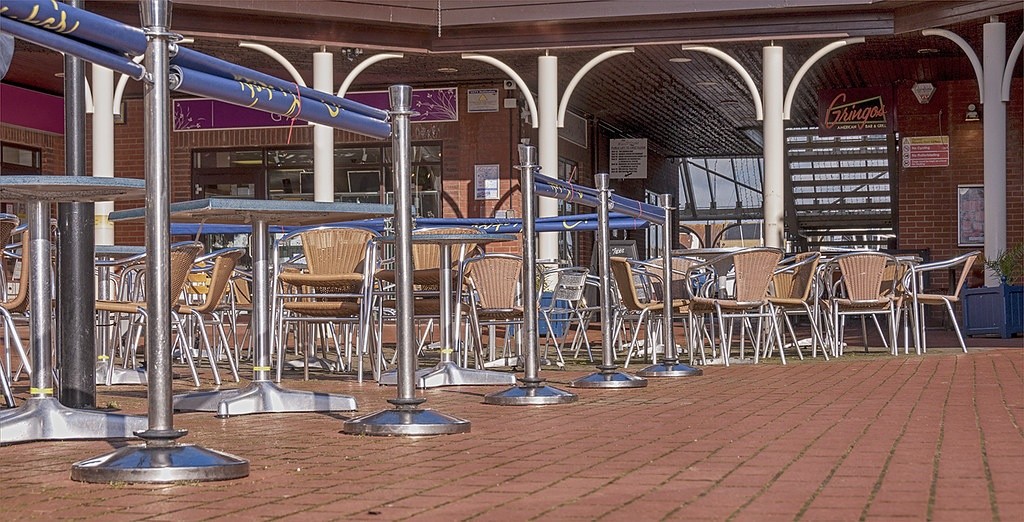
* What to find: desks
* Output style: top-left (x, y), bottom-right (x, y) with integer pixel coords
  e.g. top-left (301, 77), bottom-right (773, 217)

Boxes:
top-left (93, 246), bottom-right (148, 386)
top-left (478, 259), bottom-right (568, 370)
top-left (372, 235), bottom-right (517, 388)
top-left (0, 175), bottom-right (148, 445)
top-left (670, 247), bottom-right (787, 366)
top-left (108, 198), bottom-right (417, 421)
top-left (784, 256), bottom-right (924, 347)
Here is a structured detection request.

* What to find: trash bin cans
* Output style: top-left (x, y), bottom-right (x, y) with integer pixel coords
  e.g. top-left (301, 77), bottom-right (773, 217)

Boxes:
top-left (538, 292), bottom-right (567, 338)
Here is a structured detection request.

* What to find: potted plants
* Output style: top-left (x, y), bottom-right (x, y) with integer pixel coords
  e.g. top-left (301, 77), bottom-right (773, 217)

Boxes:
top-left (961, 239), bottom-right (1024, 339)
top-left (509, 263), bottom-right (570, 338)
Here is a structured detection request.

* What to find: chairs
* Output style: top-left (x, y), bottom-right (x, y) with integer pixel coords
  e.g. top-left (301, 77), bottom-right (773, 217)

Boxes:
top-left (0, 214), bottom-right (981, 445)
